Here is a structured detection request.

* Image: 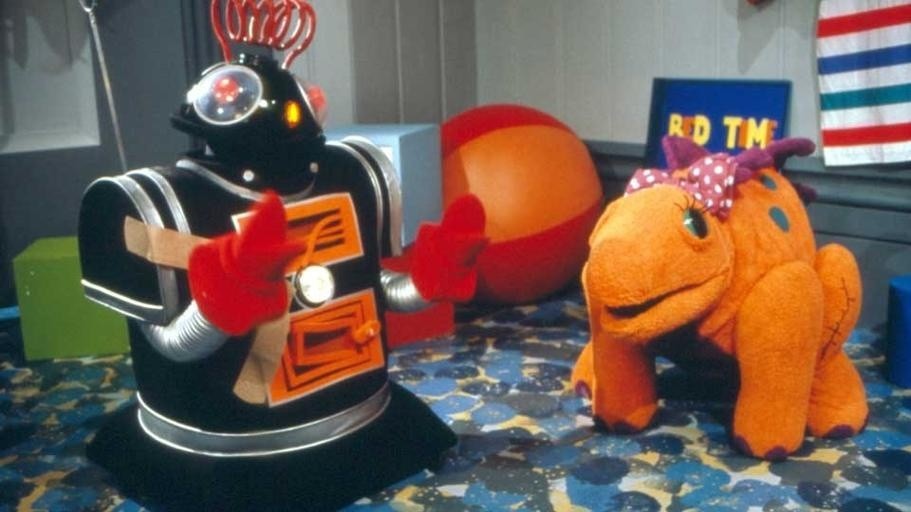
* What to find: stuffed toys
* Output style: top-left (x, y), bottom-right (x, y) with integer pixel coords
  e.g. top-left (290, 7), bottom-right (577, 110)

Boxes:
top-left (570, 136), bottom-right (869, 460)
